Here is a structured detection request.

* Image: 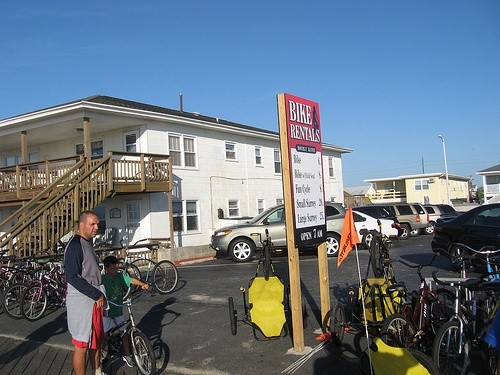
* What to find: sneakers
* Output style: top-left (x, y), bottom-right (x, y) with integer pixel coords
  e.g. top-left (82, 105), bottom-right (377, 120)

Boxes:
top-left (122, 356), bottom-right (134, 367)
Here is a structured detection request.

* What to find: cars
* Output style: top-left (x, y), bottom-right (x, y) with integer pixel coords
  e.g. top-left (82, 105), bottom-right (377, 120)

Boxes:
top-left (431, 203), bottom-right (500, 270)
top-left (343, 203), bottom-right (457, 249)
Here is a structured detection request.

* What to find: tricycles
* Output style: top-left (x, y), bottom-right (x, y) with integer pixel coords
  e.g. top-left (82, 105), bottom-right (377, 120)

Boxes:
top-left (228, 229), bottom-right (309, 341)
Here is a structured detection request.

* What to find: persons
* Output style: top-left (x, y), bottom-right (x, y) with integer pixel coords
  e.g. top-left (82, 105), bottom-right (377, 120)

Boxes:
top-left (101, 256), bottom-right (149, 367)
top-left (64, 211), bottom-right (110, 375)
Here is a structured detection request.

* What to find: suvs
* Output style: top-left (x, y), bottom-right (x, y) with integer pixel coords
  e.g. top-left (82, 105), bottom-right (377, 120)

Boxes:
top-left (209, 202), bottom-right (347, 262)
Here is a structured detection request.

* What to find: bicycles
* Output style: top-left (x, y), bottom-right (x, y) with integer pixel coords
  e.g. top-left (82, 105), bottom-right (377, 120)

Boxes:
top-left (102, 282), bottom-right (156, 375)
top-left (118, 238), bottom-right (179, 300)
top-left (0, 250), bottom-right (141, 321)
top-left (329, 219), bottom-right (500, 375)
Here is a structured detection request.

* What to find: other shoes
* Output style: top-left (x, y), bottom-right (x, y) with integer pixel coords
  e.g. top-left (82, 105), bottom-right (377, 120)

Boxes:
top-left (102, 372), bottom-right (107, 375)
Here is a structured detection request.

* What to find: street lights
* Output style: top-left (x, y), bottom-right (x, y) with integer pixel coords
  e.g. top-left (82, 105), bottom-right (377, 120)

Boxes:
top-left (438, 135), bottom-right (451, 205)
